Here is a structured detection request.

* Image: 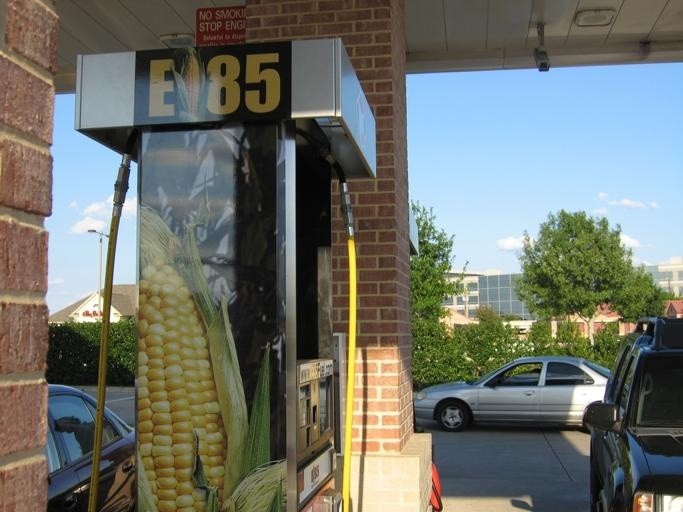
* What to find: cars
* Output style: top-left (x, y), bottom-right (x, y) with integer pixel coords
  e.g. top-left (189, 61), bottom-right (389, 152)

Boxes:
top-left (47, 384), bottom-right (137, 512)
top-left (413, 356), bottom-right (614, 434)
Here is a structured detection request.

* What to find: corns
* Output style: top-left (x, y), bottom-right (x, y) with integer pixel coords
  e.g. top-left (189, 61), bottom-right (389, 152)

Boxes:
top-left (139, 200), bottom-right (287, 512)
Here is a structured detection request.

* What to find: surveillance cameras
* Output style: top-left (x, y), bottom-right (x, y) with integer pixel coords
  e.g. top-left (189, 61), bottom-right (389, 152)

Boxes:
top-left (533, 47), bottom-right (550, 71)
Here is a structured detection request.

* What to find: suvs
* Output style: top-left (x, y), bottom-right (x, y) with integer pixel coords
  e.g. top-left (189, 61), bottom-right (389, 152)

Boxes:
top-left (580, 318), bottom-right (681, 512)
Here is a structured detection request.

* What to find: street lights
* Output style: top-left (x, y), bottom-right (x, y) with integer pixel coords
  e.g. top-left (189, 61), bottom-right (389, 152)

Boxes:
top-left (86, 228), bottom-right (109, 323)
